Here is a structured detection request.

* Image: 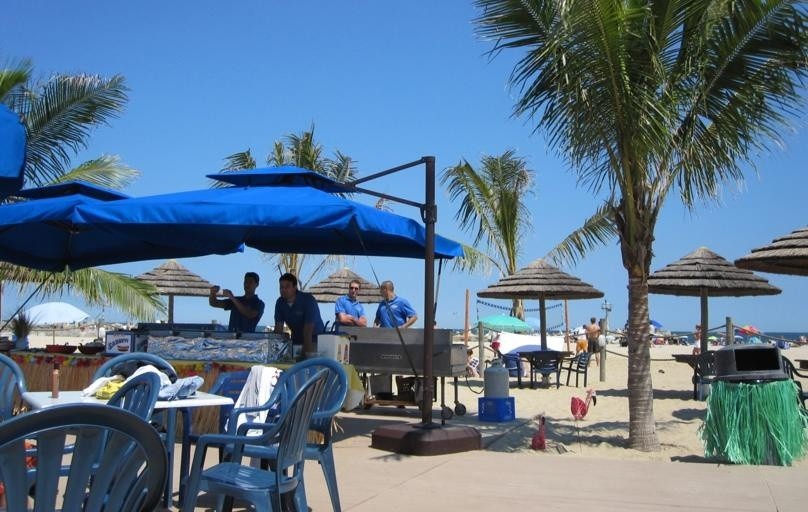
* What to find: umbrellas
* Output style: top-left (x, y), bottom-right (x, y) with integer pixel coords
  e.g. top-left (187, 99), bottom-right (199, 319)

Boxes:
top-left (474, 312), bottom-right (532, 334)
top-left (14, 302), bottom-right (89, 345)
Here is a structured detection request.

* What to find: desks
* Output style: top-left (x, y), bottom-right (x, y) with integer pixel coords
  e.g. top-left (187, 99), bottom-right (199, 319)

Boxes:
top-left (517, 349), bottom-right (575, 389)
top-left (673, 354), bottom-right (721, 379)
top-left (0, 348), bottom-right (369, 452)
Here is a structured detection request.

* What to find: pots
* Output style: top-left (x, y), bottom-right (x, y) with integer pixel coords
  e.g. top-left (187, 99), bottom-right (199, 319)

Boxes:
top-left (46, 340), bottom-right (106, 354)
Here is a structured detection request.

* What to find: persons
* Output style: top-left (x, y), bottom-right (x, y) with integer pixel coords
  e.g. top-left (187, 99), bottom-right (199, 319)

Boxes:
top-left (209, 272), bottom-right (265, 332)
top-left (574, 317), bottom-right (807, 368)
top-left (274, 273), bottom-right (325, 357)
top-left (373, 281), bottom-right (417, 409)
top-left (335, 280), bottom-right (368, 335)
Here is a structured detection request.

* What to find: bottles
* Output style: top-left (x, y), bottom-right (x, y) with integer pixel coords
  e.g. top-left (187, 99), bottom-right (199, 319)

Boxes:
top-left (51, 360), bottom-right (60, 398)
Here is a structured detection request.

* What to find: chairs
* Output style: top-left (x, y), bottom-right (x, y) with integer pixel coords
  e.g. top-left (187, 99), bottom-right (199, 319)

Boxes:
top-left (528, 349), bottom-right (563, 388)
top-left (692, 350), bottom-right (720, 401)
top-left (557, 349), bottom-right (593, 389)
top-left (1, 349), bottom-right (351, 511)
top-left (780, 354), bottom-right (808, 415)
top-left (495, 351), bottom-right (524, 387)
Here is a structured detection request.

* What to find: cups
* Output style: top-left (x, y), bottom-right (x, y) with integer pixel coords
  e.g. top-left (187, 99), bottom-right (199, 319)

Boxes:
top-left (292, 343), bottom-right (303, 358)
top-left (117, 345), bottom-right (129, 351)
top-left (304, 350), bottom-right (319, 359)
top-left (0, 336), bottom-right (16, 352)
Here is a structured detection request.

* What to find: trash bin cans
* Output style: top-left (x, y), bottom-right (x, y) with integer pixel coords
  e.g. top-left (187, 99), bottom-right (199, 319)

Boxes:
top-left (713, 344), bottom-right (792, 466)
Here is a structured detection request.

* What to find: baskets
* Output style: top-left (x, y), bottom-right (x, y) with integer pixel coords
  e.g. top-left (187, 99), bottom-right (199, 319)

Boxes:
top-left (479, 398), bottom-right (515, 422)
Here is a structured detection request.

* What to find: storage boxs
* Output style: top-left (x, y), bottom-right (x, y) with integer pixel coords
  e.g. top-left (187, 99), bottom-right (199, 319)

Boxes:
top-left (478, 396), bottom-right (516, 423)
